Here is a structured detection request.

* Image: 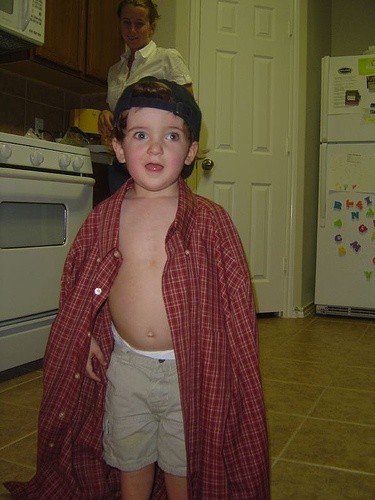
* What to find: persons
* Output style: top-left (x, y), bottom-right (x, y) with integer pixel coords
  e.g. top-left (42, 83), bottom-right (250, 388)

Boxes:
top-left (97, 0), bottom-right (195, 180)
top-left (0, 76), bottom-right (271, 500)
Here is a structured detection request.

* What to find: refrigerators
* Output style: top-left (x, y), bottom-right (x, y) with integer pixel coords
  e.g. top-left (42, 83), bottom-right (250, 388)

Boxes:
top-left (314, 52), bottom-right (375, 317)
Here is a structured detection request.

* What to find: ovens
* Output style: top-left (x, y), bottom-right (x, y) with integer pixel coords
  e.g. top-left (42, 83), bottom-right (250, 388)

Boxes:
top-left (1, 132), bottom-right (96, 373)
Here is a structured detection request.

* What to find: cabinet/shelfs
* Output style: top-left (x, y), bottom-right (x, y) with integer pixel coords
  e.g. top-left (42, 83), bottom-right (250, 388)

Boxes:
top-left (34, 1), bottom-right (124, 83)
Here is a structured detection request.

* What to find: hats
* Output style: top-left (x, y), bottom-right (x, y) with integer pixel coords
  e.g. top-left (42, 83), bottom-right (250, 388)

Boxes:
top-left (116, 76), bottom-right (202, 180)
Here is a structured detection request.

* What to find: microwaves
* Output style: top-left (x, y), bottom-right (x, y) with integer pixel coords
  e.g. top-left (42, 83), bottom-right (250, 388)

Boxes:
top-left (0, 2), bottom-right (47, 47)
